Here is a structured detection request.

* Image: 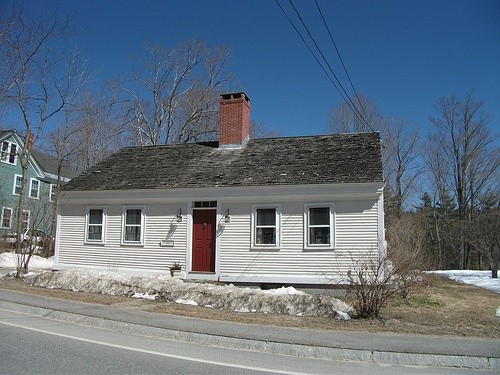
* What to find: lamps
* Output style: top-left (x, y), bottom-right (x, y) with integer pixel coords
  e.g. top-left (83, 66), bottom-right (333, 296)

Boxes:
top-left (225, 207), bottom-right (231, 222)
top-left (176, 207), bottom-right (184, 223)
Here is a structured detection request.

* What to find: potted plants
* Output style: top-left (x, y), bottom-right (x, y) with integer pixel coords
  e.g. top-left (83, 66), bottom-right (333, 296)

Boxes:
top-left (170, 260), bottom-right (182, 277)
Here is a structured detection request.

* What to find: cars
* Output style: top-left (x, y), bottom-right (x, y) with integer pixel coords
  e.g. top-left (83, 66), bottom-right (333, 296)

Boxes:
top-left (2, 227), bottom-right (56, 249)
